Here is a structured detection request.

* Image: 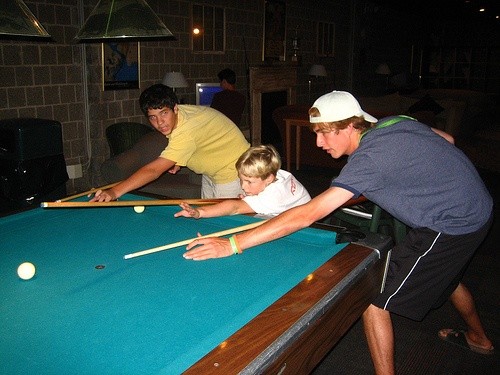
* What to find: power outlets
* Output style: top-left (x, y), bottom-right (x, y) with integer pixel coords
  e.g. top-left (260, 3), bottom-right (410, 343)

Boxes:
top-left (66, 164), bottom-right (83, 179)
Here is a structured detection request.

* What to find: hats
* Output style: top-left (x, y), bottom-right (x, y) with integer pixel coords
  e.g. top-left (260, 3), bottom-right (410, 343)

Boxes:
top-left (308, 90), bottom-right (379, 124)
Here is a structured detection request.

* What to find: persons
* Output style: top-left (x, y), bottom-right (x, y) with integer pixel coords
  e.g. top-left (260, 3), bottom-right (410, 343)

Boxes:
top-left (174, 144), bottom-right (312, 218)
top-left (182, 91), bottom-right (495, 375)
top-left (209, 69), bottom-right (246, 126)
top-left (88, 83), bottom-right (251, 203)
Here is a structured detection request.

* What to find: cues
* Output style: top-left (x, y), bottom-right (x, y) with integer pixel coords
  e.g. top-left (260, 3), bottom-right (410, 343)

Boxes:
top-left (40, 198), bottom-right (241, 207)
top-left (124, 219), bottom-right (271, 259)
top-left (54, 181), bottom-right (121, 203)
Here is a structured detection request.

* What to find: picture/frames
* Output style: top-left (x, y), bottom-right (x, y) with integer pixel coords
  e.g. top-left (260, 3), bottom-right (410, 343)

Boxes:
top-left (262, 0), bottom-right (288, 62)
top-left (102, 41), bottom-right (140, 92)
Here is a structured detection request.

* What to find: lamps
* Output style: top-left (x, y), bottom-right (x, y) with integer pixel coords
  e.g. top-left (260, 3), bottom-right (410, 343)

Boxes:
top-left (291, 32), bottom-right (301, 57)
top-left (375, 64), bottom-right (390, 74)
top-left (162, 70), bottom-right (189, 93)
top-left (0, 0), bottom-right (52, 37)
top-left (74, 0), bottom-right (175, 39)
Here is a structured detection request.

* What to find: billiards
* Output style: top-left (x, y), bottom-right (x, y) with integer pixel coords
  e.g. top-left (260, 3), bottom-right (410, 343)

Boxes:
top-left (133, 205), bottom-right (145, 214)
top-left (17, 262), bottom-right (36, 280)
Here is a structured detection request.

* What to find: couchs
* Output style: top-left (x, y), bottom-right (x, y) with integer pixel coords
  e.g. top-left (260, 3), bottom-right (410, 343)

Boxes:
top-left (271, 89), bottom-right (481, 169)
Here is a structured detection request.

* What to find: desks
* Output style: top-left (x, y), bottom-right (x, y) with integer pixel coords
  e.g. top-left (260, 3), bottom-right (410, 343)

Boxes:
top-left (0, 181), bottom-right (392, 375)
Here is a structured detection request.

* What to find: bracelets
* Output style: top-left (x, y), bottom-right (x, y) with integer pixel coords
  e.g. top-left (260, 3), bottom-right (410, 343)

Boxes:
top-left (229, 236), bottom-right (238, 254)
top-left (233, 234), bottom-right (242, 254)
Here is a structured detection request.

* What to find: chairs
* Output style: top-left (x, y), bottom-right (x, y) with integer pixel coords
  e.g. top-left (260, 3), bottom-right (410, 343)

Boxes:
top-left (106, 121), bottom-right (153, 159)
top-left (0, 118), bottom-right (65, 218)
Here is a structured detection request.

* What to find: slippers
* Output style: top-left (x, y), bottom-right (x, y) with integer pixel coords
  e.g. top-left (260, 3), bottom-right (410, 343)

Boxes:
top-left (437, 328), bottom-right (495, 356)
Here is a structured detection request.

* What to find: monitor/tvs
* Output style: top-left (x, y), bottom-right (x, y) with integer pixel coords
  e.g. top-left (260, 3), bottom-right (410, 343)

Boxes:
top-left (195, 82), bottom-right (221, 107)
